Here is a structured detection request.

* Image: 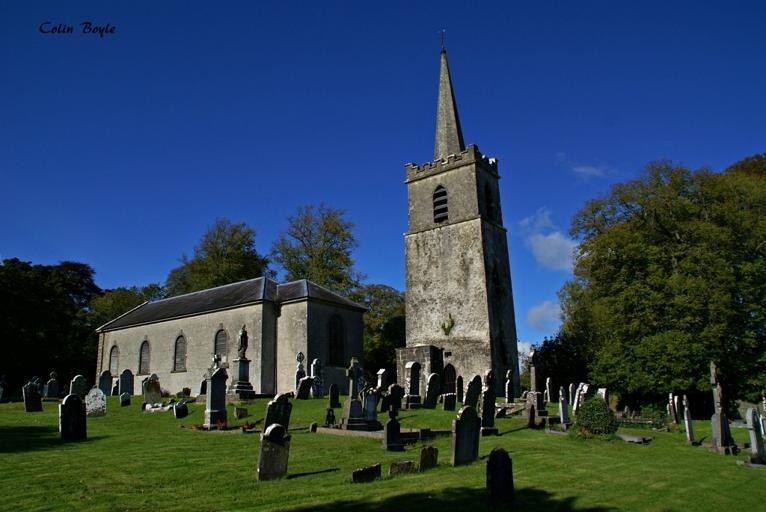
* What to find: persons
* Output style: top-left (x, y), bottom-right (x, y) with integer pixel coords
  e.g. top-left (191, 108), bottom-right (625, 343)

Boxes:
top-left (236, 323), bottom-right (248, 360)
